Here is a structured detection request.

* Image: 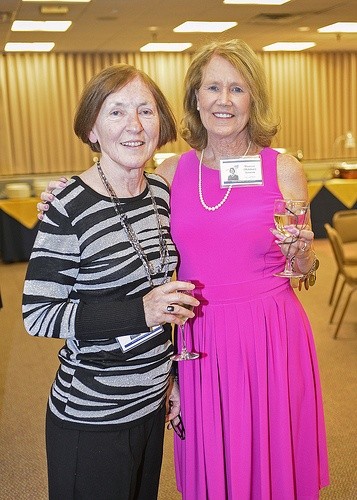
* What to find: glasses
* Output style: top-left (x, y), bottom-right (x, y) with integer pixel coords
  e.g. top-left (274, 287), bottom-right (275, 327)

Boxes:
top-left (277, 208), bottom-right (309, 259)
top-left (166, 400), bottom-right (185, 440)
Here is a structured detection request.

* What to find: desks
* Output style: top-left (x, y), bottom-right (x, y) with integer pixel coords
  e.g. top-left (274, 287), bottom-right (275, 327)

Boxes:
top-left (0, 198), bottom-right (43, 262)
top-left (308, 178), bottom-right (356, 239)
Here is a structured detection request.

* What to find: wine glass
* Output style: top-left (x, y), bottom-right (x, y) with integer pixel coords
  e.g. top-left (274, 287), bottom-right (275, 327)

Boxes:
top-left (170, 280), bottom-right (199, 361)
top-left (273, 199), bottom-right (309, 277)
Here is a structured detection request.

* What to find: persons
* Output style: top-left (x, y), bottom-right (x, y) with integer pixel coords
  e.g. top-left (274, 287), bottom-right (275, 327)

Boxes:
top-left (33, 39), bottom-right (331, 500)
top-left (20, 60), bottom-right (202, 500)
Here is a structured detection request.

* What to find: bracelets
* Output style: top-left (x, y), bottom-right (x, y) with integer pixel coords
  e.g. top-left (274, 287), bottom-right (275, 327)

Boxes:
top-left (288, 256), bottom-right (320, 291)
top-left (290, 248), bottom-right (317, 278)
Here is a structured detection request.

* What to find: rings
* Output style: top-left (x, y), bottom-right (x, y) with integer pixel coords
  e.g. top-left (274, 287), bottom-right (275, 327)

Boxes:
top-left (166, 304), bottom-right (174, 314)
top-left (302, 242), bottom-right (307, 251)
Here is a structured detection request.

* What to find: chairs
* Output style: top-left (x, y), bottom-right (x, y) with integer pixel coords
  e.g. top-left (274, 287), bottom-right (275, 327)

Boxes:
top-left (325, 209), bottom-right (357, 338)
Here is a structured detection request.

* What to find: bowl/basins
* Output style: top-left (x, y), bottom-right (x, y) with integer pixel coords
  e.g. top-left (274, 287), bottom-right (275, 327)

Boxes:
top-left (33, 182), bottom-right (47, 196)
top-left (5, 184), bottom-right (30, 199)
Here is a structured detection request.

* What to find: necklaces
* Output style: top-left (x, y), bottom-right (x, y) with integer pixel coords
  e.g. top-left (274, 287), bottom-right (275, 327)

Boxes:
top-left (96, 159), bottom-right (170, 289)
top-left (198, 138), bottom-right (252, 211)
